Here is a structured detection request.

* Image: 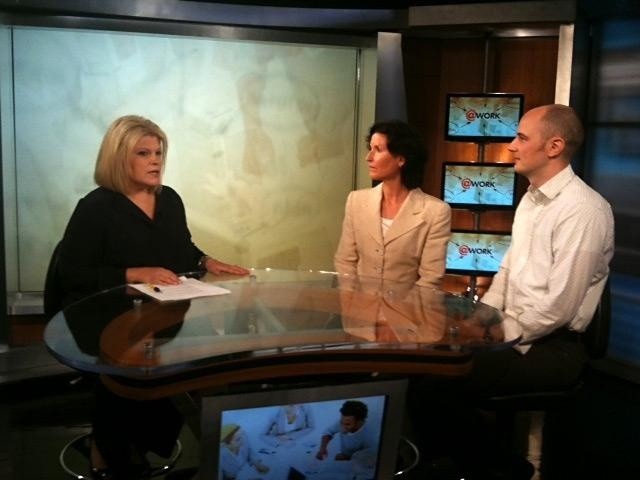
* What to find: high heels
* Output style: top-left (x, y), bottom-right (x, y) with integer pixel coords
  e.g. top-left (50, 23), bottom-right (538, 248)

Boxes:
top-left (88, 429), bottom-right (107, 479)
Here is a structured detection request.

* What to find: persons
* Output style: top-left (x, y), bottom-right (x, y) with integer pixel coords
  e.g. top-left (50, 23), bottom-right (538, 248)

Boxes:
top-left (57, 114), bottom-right (249, 479)
top-left (331, 119), bottom-right (452, 289)
top-left (258, 404), bottom-right (314, 448)
top-left (478, 104), bottom-right (615, 355)
top-left (218, 424), bottom-right (271, 479)
top-left (315, 400), bottom-right (375, 462)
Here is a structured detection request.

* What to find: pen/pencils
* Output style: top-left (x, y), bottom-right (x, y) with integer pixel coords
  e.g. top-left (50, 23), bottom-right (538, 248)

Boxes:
top-left (146, 282), bottom-right (161, 292)
top-left (175, 270), bottom-right (201, 276)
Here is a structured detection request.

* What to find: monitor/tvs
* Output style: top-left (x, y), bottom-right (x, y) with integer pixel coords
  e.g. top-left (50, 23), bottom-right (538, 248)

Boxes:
top-left (445, 228), bottom-right (512, 278)
top-left (197, 376), bottom-right (409, 480)
top-left (444, 92), bottom-right (526, 143)
top-left (440, 160), bottom-right (519, 210)
